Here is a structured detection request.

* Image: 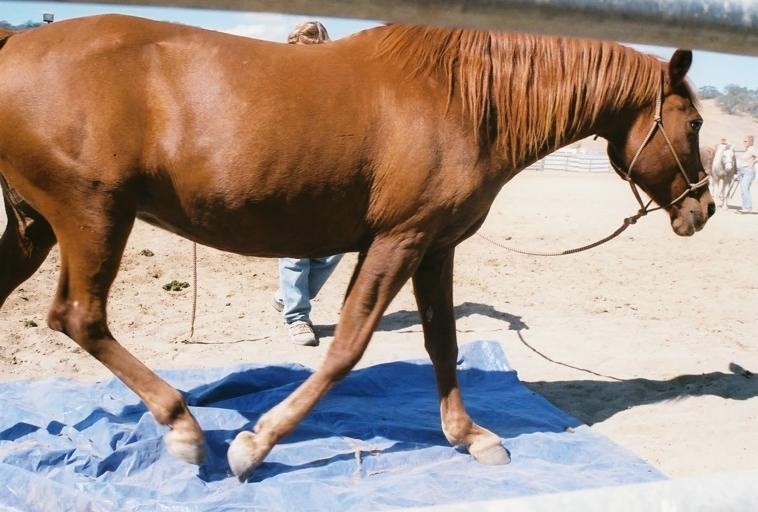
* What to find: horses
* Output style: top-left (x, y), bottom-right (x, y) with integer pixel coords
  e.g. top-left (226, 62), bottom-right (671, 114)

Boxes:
top-left (711, 143), bottom-right (736, 210)
top-left (0, 14), bottom-right (716, 484)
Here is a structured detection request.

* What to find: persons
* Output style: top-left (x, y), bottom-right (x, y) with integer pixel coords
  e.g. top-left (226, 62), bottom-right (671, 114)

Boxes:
top-left (268, 19), bottom-right (347, 348)
top-left (735, 136), bottom-right (758, 214)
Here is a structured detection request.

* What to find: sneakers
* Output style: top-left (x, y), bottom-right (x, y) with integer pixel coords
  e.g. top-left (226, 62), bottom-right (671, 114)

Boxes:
top-left (271, 301), bottom-right (285, 312)
top-left (288, 324), bottom-right (317, 346)
